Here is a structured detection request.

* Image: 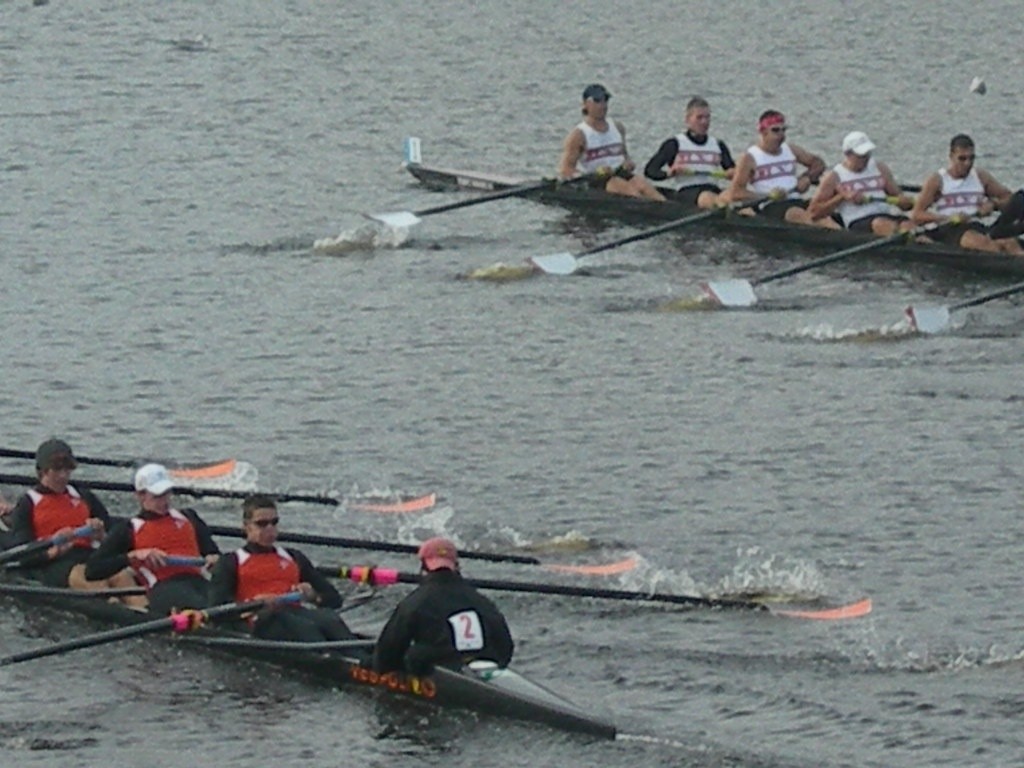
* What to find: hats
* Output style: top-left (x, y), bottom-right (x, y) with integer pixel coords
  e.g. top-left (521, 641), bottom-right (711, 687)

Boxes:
top-left (134, 464), bottom-right (178, 495)
top-left (842, 131), bottom-right (875, 156)
top-left (418, 538), bottom-right (457, 570)
top-left (584, 84), bottom-right (613, 98)
top-left (35, 438), bottom-right (79, 468)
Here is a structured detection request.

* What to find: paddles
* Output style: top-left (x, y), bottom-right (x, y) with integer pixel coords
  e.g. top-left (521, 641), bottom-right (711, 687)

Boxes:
top-left (362, 168), bottom-right (598, 226)
top-left (709, 209), bottom-right (979, 306)
top-left (1, 518), bottom-right (107, 567)
top-left (2, 592), bottom-right (300, 668)
top-left (2, 446), bottom-right (238, 477)
top-left (908, 281), bottom-right (1024, 334)
top-left (210, 524), bottom-right (636, 573)
top-left (532, 186), bottom-right (798, 276)
top-left (675, 164), bottom-right (925, 194)
top-left (311, 563), bottom-right (873, 621)
top-left (861, 193), bottom-right (915, 206)
top-left (2, 472), bottom-right (438, 513)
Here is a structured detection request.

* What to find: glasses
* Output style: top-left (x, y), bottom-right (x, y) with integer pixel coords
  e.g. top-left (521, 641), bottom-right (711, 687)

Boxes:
top-left (766, 127), bottom-right (786, 132)
top-left (590, 96), bottom-right (608, 103)
top-left (248, 518), bottom-right (278, 526)
top-left (953, 153), bottom-right (976, 161)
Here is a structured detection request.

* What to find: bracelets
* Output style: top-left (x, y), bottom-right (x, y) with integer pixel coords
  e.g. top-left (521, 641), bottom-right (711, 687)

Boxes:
top-left (989, 197), bottom-right (1000, 211)
top-left (803, 170), bottom-right (815, 180)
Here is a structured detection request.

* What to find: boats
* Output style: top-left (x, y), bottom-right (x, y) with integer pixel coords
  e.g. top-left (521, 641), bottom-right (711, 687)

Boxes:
top-left (1, 504), bottom-right (618, 743)
top-left (403, 136), bottom-right (1024, 278)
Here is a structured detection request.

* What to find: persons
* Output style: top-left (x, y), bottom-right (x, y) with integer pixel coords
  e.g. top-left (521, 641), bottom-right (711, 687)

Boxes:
top-left (560, 84), bottom-right (668, 201)
top-left (0, 490), bottom-right (13, 518)
top-left (912, 133), bottom-right (1024, 255)
top-left (207, 496), bottom-right (369, 657)
top-left (645, 96), bottom-right (736, 209)
top-left (359, 537), bottom-right (514, 678)
top-left (730, 110), bottom-right (843, 230)
top-left (807, 131), bottom-right (915, 235)
top-left (84, 463), bottom-right (222, 612)
top-left (11, 438), bottom-right (150, 607)
